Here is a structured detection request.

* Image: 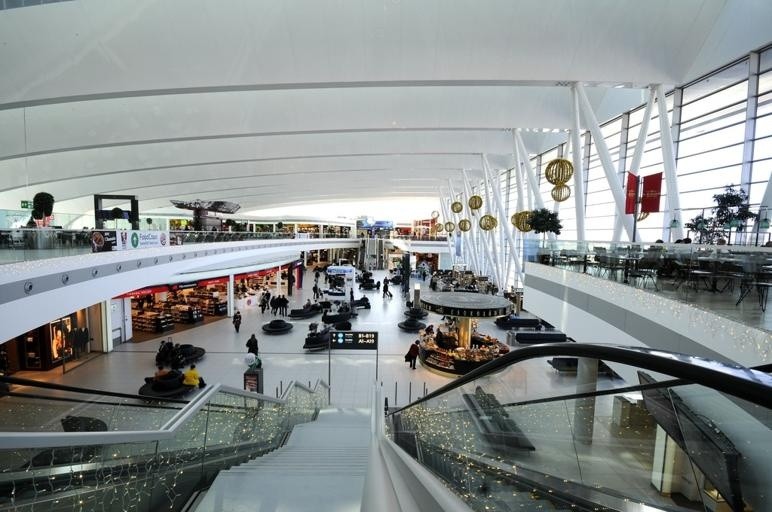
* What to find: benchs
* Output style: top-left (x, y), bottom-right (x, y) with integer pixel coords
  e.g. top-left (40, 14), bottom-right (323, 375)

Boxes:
top-left (473, 383), bottom-right (536, 458)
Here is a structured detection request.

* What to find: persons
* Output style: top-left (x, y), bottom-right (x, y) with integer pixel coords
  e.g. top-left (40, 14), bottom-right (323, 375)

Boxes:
top-left (636, 237), bottom-right (731, 279)
top-left (432, 274), bottom-right (440, 290)
top-left (158, 341), bottom-right (168, 355)
top-left (172, 343), bottom-right (182, 363)
top-left (233, 311), bottom-right (242, 332)
top-left (304, 270), bottom-right (393, 313)
top-left (435, 328), bottom-right (444, 348)
top-left (422, 271), bottom-right (427, 280)
top-left (155, 366), bottom-right (168, 380)
top-left (409, 339), bottom-right (421, 370)
top-left (246, 333), bottom-right (259, 356)
top-left (423, 324), bottom-right (433, 341)
top-left (183, 364), bottom-right (206, 389)
top-left (80, 327), bottom-right (90, 356)
top-left (69, 327), bottom-right (81, 362)
top-left (259, 290), bottom-right (289, 317)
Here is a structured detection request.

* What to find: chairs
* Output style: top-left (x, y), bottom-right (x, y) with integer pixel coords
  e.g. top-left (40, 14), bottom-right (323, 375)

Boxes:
top-left (540, 244), bottom-right (771, 313)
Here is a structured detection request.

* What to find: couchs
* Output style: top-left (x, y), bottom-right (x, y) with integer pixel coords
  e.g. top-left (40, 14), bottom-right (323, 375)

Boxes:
top-left (288, 296), bottom-right (355, 352)
top-left (137, 342), bottom-right (206, 399)
top-left (261, 318), bottom-right (294, 334)
top-left (398, 305), bottom-right (428, 332)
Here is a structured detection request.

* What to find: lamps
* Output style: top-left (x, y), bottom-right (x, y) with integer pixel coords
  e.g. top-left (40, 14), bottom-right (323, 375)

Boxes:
top-left (434, 128), bottom-right (574, 236)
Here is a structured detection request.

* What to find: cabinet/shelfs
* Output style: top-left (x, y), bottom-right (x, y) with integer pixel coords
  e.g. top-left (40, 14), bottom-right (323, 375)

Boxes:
top-left (128, 272), bottom-right (278, 334)
